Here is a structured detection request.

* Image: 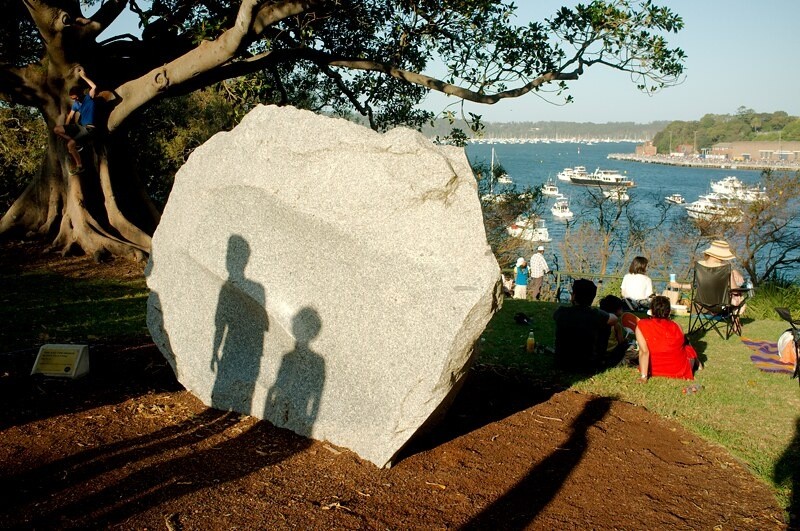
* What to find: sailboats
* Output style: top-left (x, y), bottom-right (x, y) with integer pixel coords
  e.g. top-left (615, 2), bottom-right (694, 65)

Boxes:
top-left (471, 132), bottom-right (644, 146)
top-left (480, 148), bottom-right (533, 203)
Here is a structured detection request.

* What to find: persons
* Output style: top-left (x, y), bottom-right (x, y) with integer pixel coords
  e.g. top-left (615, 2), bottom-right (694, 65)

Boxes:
top-left (635, 296), bottom-right (702, 383)
top-left (54, 70), bottom-right (97, 174)
top-left (667, 240), bottom-right (745, 315)
top-left (552, 278), bottom-right (625, 372)
top-left (621, 256), bottom-right (653, 302)
top-left (514, 258), bottom-right (531, 300)
top-left (530, 247), bottom-right (550, 301)
top-left (503, 274), bottom-right (514, 289)
top-left (599, 295), bottom-right (623, 352)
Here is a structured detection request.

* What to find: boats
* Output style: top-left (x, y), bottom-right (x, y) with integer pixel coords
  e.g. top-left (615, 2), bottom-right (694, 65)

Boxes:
top-left (603, 187), bottom-right (630, 200)
top-left (686, 177), bottom-right (770, 224)
top-left (505, 215), bottom-right (552, 243)
top-left (551, 201), bottom-right (574, 218)
top-left (542, 173), bottom-right (559, 195)
top-left (664, 194), bottom-right (685, 204)
top-left (497, 173), bottom-right (512, 184)
top-left (558, 165), bottom-right (634, 188)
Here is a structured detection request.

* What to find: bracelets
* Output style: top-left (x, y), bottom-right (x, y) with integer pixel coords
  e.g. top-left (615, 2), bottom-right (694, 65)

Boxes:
top-left (641, 375), bottom-right (648, 378)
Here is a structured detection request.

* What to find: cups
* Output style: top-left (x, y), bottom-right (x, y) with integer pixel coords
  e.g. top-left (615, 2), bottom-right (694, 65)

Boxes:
top-left (670, 274), bottom-right (676, 290)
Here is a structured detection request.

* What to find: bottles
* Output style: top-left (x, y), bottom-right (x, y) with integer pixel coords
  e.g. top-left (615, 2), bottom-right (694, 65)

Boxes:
top-left (525, 329), bottom-right (535, 352)
top-left (682, 385), bottom-right (701, 394)
top-left (631, 336), bottom-right (635, 345)
top-left (746, 281), bottom-right (755, 297)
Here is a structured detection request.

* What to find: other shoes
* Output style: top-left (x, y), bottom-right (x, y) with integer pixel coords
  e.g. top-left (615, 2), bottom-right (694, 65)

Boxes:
top-left (69, 165), bottom-right (85, 175)
top-left (69, 145), bottom-right (84, 153)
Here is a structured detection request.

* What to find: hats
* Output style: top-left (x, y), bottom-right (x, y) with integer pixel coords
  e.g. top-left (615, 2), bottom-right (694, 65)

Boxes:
top-left (538, 246), bottom-right (544, 250)
top-left (704, 240), bottom-right (737, 260)
top-left (516, 257), bottom-right (526, 267)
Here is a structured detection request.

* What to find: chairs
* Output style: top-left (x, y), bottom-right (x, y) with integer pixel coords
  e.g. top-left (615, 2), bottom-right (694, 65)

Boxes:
top-left (688, 258), bottom-right (754, 341)
